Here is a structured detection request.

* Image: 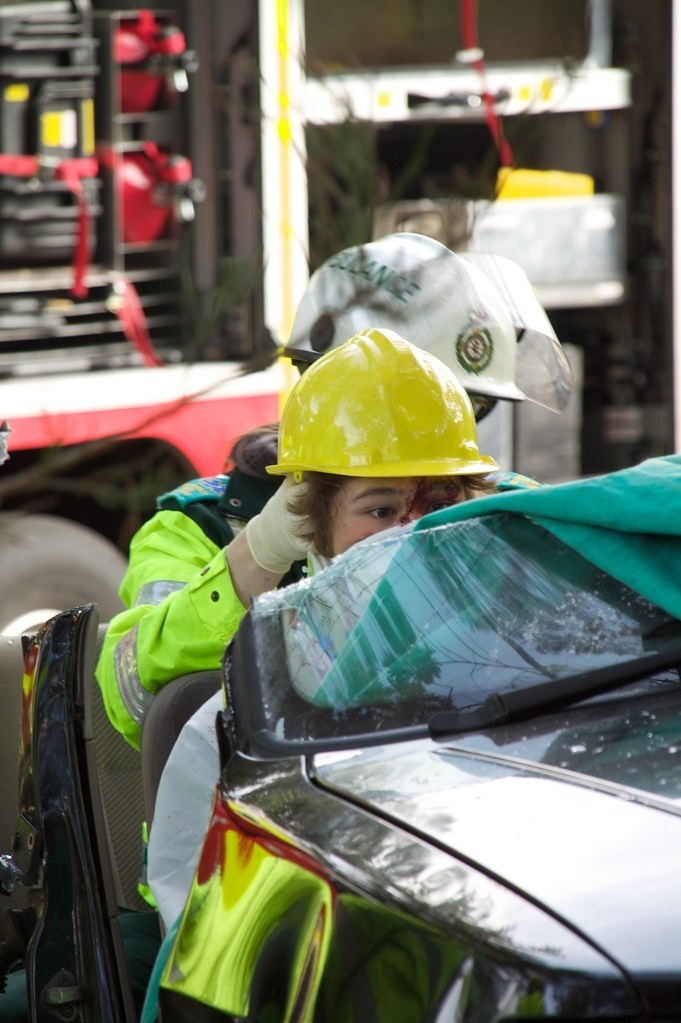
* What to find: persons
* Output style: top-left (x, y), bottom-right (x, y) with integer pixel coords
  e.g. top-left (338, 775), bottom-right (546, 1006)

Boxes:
top-left (90, 225), bottom-right (586, 920)
top-left (144, 330), bottom-right (504, 1023)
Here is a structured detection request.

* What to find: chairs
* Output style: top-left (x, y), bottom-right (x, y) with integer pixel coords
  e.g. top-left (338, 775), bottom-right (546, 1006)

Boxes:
top-left (17, 603), bottom-right (159, 1023)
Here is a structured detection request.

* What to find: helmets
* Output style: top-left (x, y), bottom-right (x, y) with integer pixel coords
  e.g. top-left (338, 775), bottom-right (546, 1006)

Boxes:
top-left (279, 228), bottom-right (574, 416)
top-left (262, 327), bottom-right (497, 489)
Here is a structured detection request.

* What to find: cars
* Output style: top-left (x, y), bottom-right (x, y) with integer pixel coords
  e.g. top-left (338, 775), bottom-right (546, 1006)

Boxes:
top-left (157, 672), bottom-right (680, 1023)
